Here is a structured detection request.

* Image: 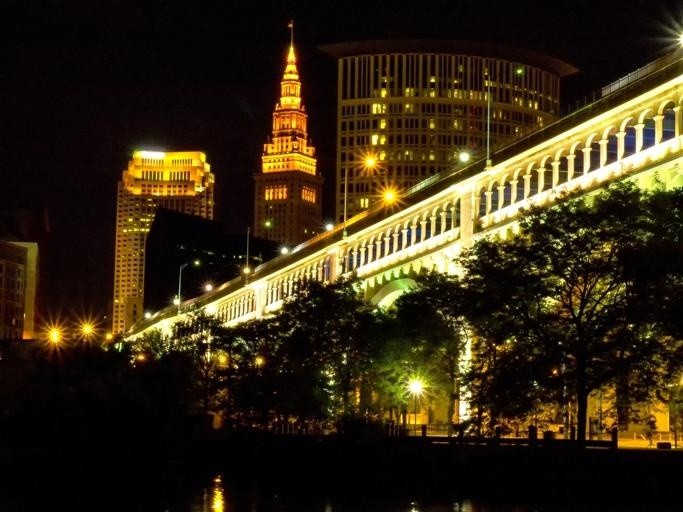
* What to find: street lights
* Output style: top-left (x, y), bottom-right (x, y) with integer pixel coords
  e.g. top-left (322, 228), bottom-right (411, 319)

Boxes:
top-left (175, 257), bottom-right (202, 315)
top-left (408, 379), bottom-right (423, 438)
top-left (481, 65), bottom-right (528, 170)
top-left (342, 154), bottom-right (383, 238)
top-left (244, 219), bottom-right (274, 285)
